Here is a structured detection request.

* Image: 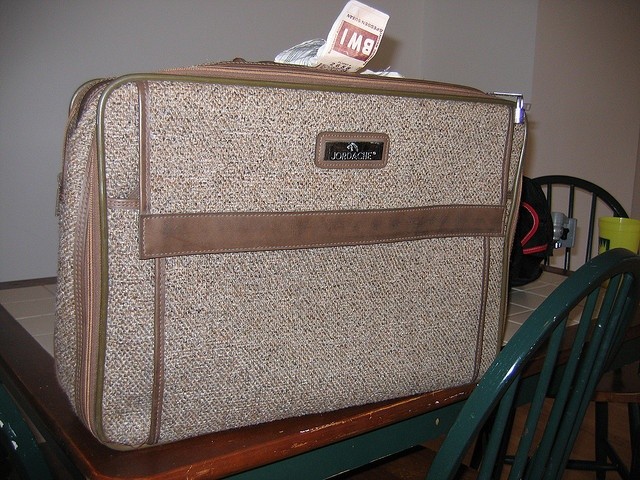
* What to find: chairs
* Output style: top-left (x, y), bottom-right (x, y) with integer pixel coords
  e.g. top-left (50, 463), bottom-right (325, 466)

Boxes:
top-left (328, 248), bottom-right (640, 479)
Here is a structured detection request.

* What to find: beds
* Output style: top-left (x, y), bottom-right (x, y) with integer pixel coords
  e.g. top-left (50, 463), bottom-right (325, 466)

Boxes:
top-left (0, 173), bottom-right (633, 480)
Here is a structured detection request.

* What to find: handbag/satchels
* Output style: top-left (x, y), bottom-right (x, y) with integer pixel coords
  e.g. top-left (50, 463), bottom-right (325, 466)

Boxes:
top-left (53, 58), bottom-right (529, 453)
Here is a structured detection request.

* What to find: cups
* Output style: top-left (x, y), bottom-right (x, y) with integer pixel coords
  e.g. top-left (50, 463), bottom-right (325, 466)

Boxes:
top-left (598, 216), bottom-right (640, 290)
